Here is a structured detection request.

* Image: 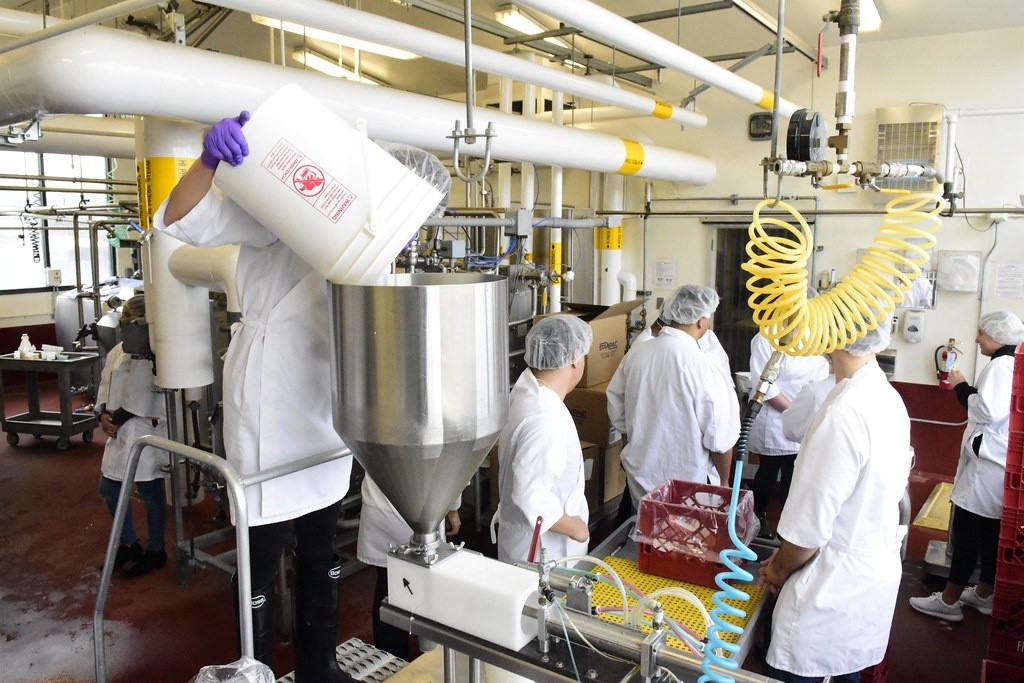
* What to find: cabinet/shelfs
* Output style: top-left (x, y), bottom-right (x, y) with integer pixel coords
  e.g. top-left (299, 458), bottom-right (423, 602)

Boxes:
top-left (0, 350), bottom-right (103, 450)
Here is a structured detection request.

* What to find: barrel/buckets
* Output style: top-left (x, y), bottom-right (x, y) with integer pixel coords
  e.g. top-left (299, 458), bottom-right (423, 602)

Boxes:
top-left (212, 81), bottom-right (449, 286)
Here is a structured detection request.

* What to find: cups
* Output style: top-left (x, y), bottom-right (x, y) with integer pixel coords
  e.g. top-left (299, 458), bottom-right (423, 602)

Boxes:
top-left (56, 355), bottom-right (68, 360)
top-left (32, 352), bottom-right (39, 360)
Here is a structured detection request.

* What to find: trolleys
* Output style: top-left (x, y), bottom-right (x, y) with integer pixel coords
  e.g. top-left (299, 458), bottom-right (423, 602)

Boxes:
top-left (0, 350), bottom-right (102, 450)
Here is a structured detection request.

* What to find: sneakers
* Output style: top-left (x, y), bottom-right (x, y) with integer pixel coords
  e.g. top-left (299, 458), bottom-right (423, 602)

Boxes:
top-left (961, 585), bottom-right (993, 614)
top-left (909, 593), bottom-right (965, 622)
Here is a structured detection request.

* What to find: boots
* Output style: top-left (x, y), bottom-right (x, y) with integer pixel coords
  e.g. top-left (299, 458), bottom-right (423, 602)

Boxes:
top-left (290, 546), bottom-right (357, 683)
top-left (229, 568), bottom-right (274, 666)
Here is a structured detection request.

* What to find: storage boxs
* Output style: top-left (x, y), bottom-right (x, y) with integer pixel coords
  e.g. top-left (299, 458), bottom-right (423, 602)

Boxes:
top-left (490, 298), bottom-right (650, 521)
top-left (981, 341), bottom-right (1024, 683)
top-left (859, 641), bottom-right (893, 683)
top-left (639, 479), bottom-right (752, 590)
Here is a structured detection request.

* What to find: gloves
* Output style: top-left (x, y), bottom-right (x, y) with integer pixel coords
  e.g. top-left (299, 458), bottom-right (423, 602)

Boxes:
top-left (201, 110), bottom-right (251, 170)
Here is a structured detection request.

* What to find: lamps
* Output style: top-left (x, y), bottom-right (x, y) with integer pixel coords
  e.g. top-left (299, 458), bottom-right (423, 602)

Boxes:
top-left (292, 47), bottom-right (387, 87)
top-left (495, 3), bottom-right (588, 70)
top-left (250, 13), bottom-right (423, 61)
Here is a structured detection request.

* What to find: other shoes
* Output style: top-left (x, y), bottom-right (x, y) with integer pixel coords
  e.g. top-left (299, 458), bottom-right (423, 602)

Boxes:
top-left (754, 511), bottom-right (773, 540)
top-left (100, 544), bottom-right (144, 570)
top-left (125, 547), bottom-right (167, 579)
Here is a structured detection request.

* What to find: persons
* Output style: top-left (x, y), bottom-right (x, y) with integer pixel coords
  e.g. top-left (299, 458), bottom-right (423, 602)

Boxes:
top-left (749, 285), bottom-right (830, 539)
top-left (356, 467), bottom-right (470, 661)
top-left (153, 110), bottom-right (451, 683)
top-left (491, 314), bottom-right (593, 568)
top-left (93, 294), bottom-right (171, 578)
top-left (909, 311), bottom-right (1024, 620)
top-left (606, 284), bottom-right (741, 529)
top-left (759, 297), bottom-right (911, 683)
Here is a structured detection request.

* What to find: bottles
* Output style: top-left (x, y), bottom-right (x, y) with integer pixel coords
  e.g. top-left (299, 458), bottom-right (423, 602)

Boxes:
top-left (18, 334), bottom-right (33, 359)
top-left (821, 268), bottom-right (830, 289)
